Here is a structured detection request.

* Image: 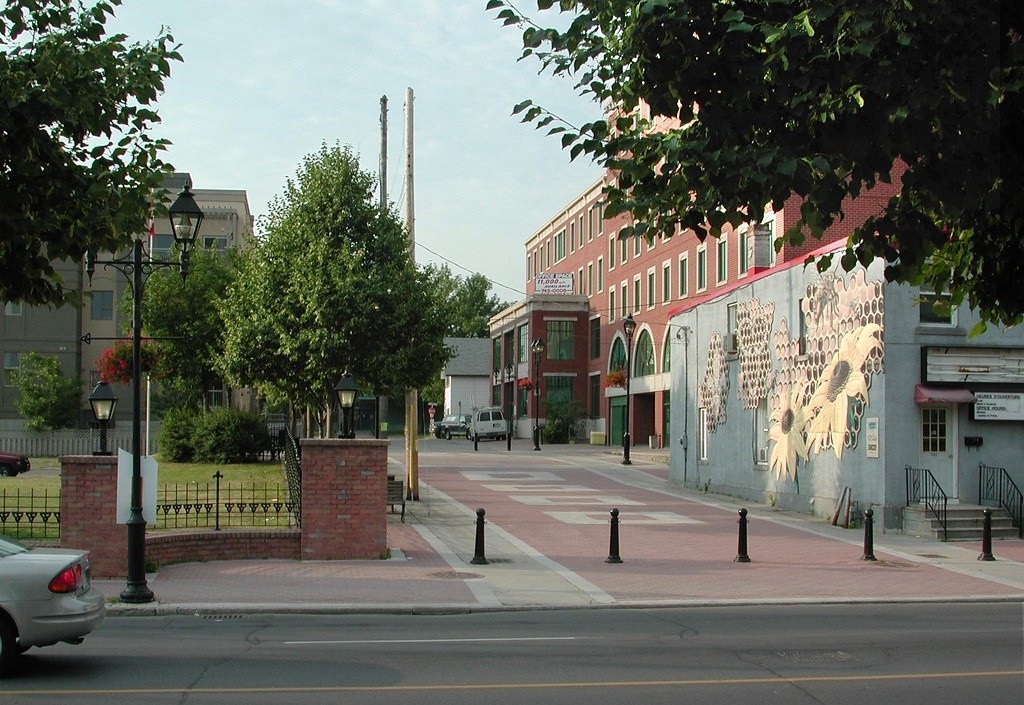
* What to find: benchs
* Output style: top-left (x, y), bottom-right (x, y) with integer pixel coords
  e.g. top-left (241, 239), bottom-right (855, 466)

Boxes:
top-left (388, 480), bottom-right (406, 523)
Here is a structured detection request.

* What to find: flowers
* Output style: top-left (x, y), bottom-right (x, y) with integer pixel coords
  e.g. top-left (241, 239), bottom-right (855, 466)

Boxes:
top-left (601, 369), bottom-right (628, 390)
top-left (518, 379), bottom-right (533, 392)
top-left (91, 331), bottom-right (163, 387)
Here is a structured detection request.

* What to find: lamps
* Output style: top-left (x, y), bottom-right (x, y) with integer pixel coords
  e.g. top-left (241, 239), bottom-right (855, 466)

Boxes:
top-left (87, 380), bottom-right (119, 456)
top-left (334, 369), bottom-right (360, 439)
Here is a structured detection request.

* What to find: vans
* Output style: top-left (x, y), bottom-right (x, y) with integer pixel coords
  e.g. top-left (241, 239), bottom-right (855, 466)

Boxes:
top-left (466, 406), bottom-right (508, 442)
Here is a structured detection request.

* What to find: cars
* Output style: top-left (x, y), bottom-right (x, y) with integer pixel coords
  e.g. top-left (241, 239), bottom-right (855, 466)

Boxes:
top-left (0, 534), bottom-right (108, 669)
top-left (0, 449), bottom-right (32, 478)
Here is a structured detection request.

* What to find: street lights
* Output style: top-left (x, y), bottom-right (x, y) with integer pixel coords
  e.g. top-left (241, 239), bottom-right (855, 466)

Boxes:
top-left (530, 337), bottom-right (544, 451)
top-left (83, 182), bottom-right (205, 607)
top-left (622, 313), bottom-right (637, 465)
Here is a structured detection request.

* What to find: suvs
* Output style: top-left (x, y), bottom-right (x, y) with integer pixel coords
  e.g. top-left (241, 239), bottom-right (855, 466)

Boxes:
top-left (431, 414), bottom-right (470, 441)
